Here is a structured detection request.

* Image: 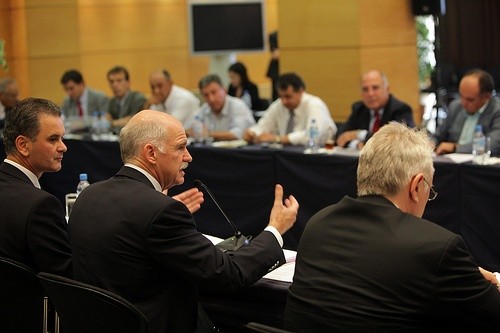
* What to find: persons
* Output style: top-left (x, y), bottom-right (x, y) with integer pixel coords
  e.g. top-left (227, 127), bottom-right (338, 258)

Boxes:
top-left (243, 74), bottom-right (337, 148)
top-left (188, 74), bottom-right (256, 142)
top-left (336, 69), bottom-right (413, 151)
top-left (431, 70), bottom-right (500, 155)
top-left (105, 67), bottom-right (146, 131)
top-left (68, 110), bottom-right (299, 333)
top-left (228, 63), bottom-right (259, 111)
top-left (287, 121), bottom-right (500, 333)
top-left (0, 80), bottom-right (19, 121)
top-left (0, 97), bottom-right (71, 269)
top-left (144, 68), bottom-right (201, 133)
top-left (61, 70), bottom-right (108, 116)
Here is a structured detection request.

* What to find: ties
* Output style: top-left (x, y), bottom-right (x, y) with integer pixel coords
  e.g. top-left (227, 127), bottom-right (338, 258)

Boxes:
top-left (76, 100), bottom-right (84, 117)
top-left (371, 111), bottom-right (380, 133)
top-left (285, 109), bottom-right (296, 135)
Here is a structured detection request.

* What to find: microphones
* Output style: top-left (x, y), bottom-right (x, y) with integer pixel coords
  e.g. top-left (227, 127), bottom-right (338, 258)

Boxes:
top-left (192, 179), bottom-right (248, 253)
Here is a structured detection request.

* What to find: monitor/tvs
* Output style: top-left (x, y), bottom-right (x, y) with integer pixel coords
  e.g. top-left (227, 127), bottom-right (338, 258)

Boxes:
top-left (189, 0), bottom-right (270, 56)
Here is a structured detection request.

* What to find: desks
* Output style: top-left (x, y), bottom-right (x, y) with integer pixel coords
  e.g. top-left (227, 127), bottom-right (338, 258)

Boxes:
top-left (42, 133), bottom-right (500, 273)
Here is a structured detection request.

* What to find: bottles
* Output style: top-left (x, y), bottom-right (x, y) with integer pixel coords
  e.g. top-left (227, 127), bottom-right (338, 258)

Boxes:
top-left (92, 111), bottom-right (110, 141)
top-left (472, 124), bottom-right (486, 159)
top-left (308, 119), bottom-right (319, 149)
top-left (325, 126), bottom-right (334, 148)
top-left (194, 116), bottom-right (204, 143)
top-left (76, 173), bottom-right (91, 198)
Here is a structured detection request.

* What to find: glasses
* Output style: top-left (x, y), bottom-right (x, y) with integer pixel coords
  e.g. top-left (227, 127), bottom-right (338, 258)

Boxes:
top-left (411, 174), bottom-right (438, 200)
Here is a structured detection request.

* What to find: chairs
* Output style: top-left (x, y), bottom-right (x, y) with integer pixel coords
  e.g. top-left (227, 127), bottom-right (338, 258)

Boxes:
top-left (0, 256), bottom-right (290, 333)
